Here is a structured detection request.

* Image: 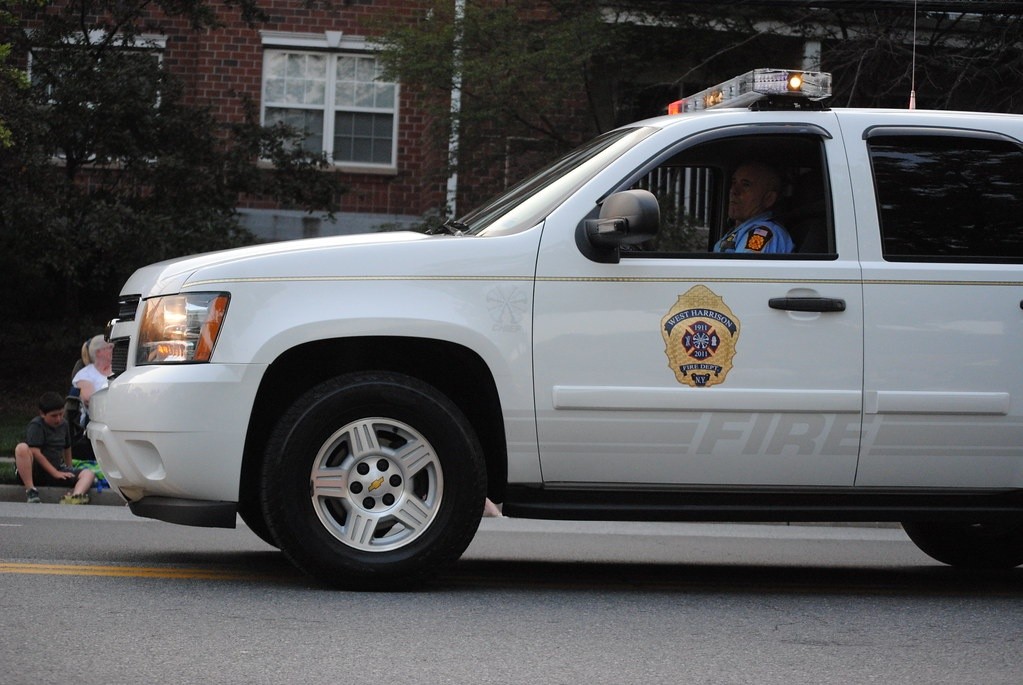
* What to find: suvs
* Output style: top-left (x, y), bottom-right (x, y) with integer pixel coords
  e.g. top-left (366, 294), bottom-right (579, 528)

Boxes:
top-left (86, 67), bottom-right (1023, 596)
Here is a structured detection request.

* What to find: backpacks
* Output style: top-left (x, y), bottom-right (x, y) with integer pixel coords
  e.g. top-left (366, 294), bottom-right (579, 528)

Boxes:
top-left (64, 458), bottom-right (110, 492)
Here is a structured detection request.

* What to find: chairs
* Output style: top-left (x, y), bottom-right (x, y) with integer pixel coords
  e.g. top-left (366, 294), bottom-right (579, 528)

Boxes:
top-left (778, 177), bottom-right (827, 253)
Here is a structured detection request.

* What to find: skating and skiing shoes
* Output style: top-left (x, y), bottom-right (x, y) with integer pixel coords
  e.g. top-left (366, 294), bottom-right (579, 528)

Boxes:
top-left (59, 493), bottom-right (89, 505)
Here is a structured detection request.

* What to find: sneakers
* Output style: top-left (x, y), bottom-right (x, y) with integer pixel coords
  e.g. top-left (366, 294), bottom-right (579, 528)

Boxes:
top-left (24, 487), bottom-right (39, 503)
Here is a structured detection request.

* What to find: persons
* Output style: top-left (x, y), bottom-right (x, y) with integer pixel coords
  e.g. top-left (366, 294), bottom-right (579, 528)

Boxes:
top-left (712, 160), bottom-right (797, 253)
top-left (14, 334), bottom-right (114, 505)
